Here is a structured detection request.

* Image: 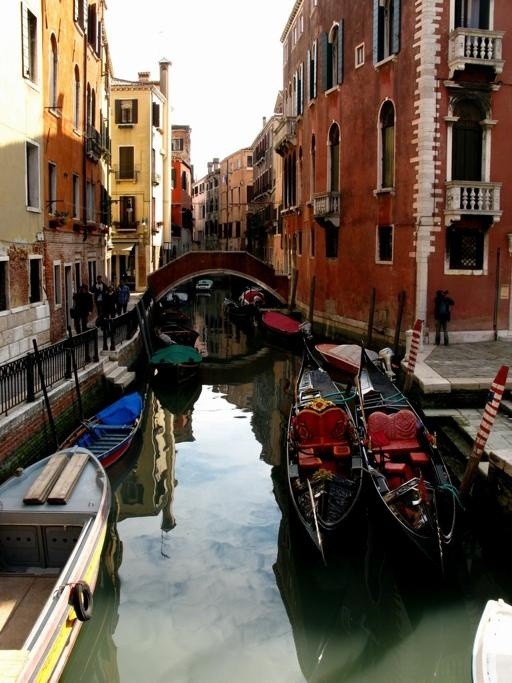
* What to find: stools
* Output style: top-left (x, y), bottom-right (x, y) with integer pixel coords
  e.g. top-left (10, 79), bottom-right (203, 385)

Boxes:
top-left (294, 445), bottom-right (352, 482)
top-left (375, 451), bottom-right (430, 466)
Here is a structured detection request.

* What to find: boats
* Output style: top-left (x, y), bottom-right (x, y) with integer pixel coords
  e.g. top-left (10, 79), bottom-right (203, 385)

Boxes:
top-left (197, 276), bottom-right (212, 291)
top-left (151, 287), bottom-right (202, 389)
top-left (56, 393), bottom-right (145, 467)
top-left (223, 285), bottom-right (308, 343)
top-left (1, 445), bottom-right (125, 683)
top-left (354, 345), bottom-right (460, 570)
top-left (285, 338), bottom-right (364, 565)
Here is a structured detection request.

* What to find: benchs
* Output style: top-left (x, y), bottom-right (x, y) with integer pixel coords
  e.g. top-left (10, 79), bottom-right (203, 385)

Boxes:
top-left (292, 406), bottom-right (348, 449)
top-left (367, 407), bottom-right (419, 453)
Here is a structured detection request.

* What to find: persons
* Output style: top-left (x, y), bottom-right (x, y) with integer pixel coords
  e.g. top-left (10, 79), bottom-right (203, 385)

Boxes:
top-left (89, 274), bottom-right (131, 338)
top-left (70, 283), bottom-right (94, 348)
top-left (432, 289), bottom-right (457, 346)
